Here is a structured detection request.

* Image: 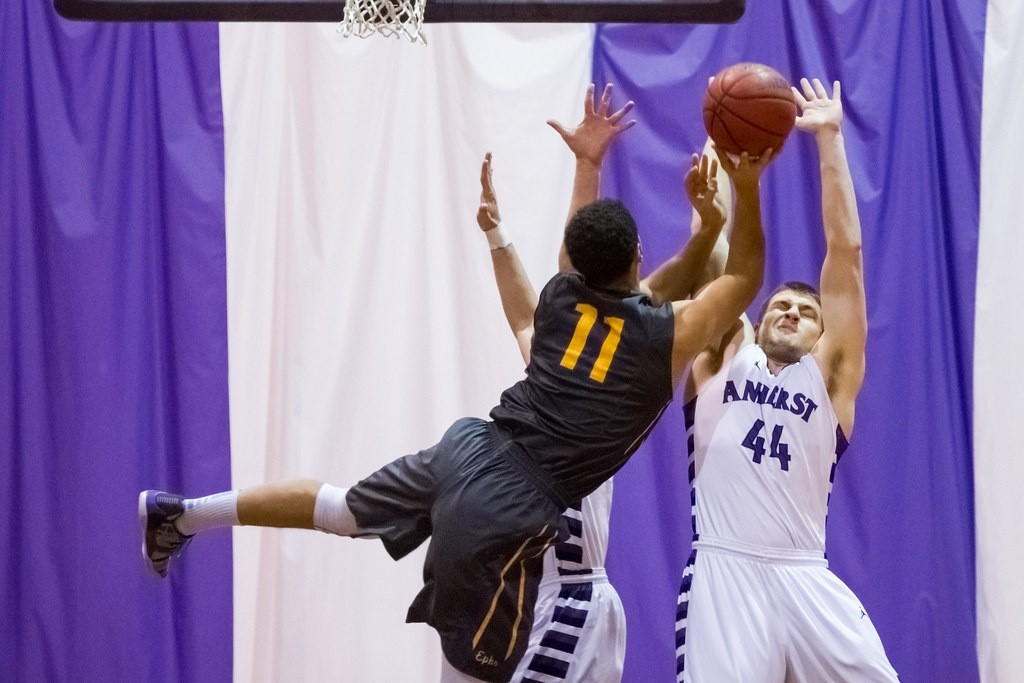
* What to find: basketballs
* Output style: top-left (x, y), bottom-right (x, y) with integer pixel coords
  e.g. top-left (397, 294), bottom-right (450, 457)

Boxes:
top-left (703, 60), bottom-right (797, 158)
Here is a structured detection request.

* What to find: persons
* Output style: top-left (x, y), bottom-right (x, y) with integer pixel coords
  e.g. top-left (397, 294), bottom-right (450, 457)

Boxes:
top-left (138, 84), bottom-right (786, 683)
top-left (674, 80), bottom-right (899, 683)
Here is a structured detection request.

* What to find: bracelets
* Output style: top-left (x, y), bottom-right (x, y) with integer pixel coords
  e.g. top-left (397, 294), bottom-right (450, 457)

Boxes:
top-left (485, 220), bottom-right (512, 250)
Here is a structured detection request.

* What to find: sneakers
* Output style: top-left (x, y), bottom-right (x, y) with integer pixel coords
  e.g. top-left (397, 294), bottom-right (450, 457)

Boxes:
top-left (139, 489), bottom-right (195, 578)
